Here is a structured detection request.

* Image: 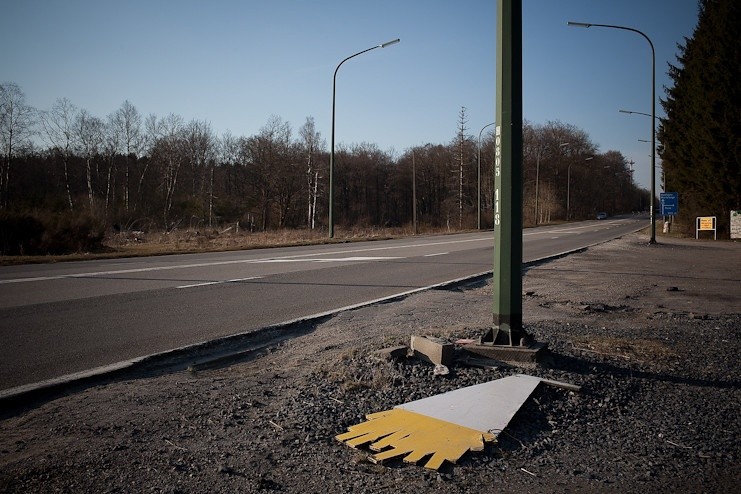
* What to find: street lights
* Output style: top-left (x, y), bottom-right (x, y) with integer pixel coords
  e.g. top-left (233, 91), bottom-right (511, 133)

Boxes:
top-left (567, 22), bottom-right (658, 244)
top-left (567, 157), bottom-right (593, 222)
top-left (535, 143), bottom-right (569, 226)
top-left (619, 110), bottom-right (665, 222)
top-left (329, 39), bottom-right (400, 238)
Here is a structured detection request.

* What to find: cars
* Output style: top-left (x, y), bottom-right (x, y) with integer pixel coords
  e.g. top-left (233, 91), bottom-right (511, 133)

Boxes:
top-left (596, 212), bottom-right (608, 220)
